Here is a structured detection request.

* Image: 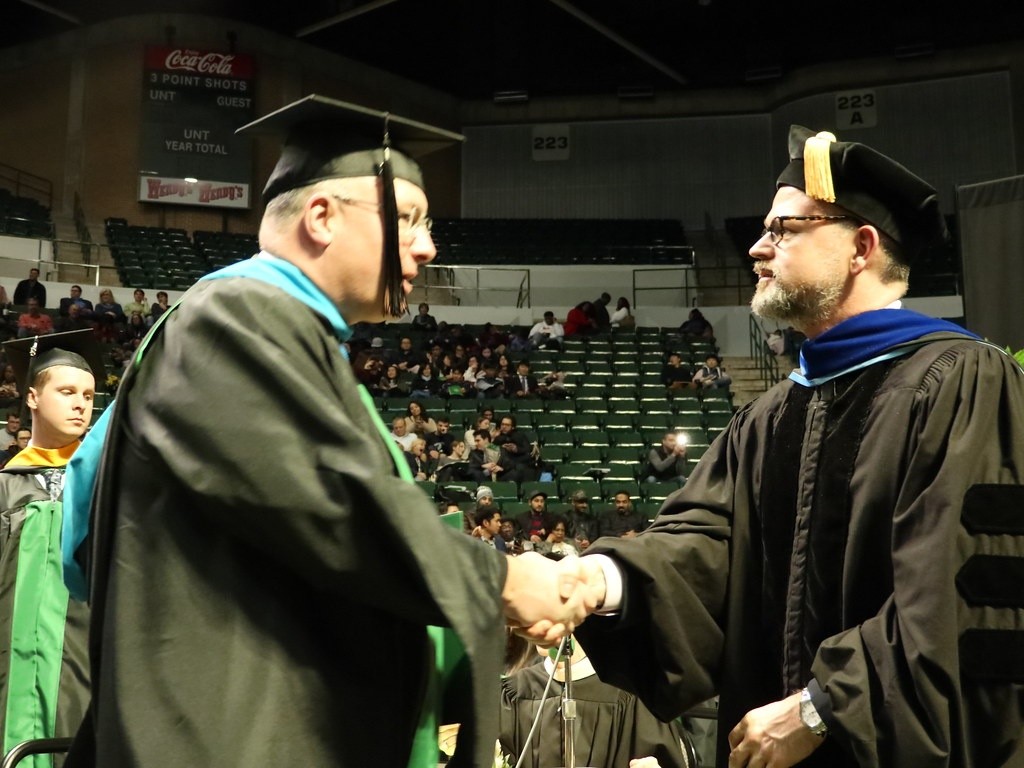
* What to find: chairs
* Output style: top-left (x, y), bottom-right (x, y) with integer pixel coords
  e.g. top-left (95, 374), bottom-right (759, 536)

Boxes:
top-left (728, 212), bottom-right (765, 284)
top-left (0, 188), bottom-right (53, 240)
top-left (105, 217), bottom-right (260, 293)
top-left (351, 321), bottom-right (734, 518)
top-left (430, 216), bottom-right (695, 266)
top-left (0, 306), bottom-right (134, 428)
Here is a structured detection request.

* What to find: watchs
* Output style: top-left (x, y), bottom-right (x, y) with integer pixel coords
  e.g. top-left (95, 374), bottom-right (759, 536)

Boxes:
top-left (799, 685), bottom-right (828, 736)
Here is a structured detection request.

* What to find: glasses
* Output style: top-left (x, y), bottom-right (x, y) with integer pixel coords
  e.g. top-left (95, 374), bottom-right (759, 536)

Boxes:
top-left (483, 414), bottom-right (492, 416)
top-left (760, 215), bottom-right (865, 246)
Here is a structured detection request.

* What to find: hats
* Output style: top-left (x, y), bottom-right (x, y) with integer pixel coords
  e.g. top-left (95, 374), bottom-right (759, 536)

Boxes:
top-left (774, 124), bottom-right (951, 272)
top-left (475, 484), bottom-right (495, 506)
top-left (370, 337), bottom-right (383, 349)
top-left (529, 489), bottom-right (549, 500)
top-left (235, 94), bottom-right (465, 205)
top-left (0, 328), bottom-right (109, 398)
top-left (573, 489), bottom-right (588, 501)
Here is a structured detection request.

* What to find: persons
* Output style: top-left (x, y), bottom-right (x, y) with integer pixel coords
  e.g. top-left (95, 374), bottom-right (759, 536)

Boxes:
top-left (63, 93), bottom-right (597, 768)
top-left (639, 430), bottom-right (690, 504)
top-left (0, 428), bottom-right (31, 470)
top-left (0, 347), bottom-right (96, 768)
top-left (124, 289), bottom-right (151, 317)
top-left (514, 490), bottom-right (556, 543)
top-left (152, 291), bottom-right (172, 323)
top-left (404, 401), bottom-right (436, 433)
top-left (599, 489), bottom-right (650, 537)
top-left (502, 124), bottom-right (1023, 768)
top-left (0, 269), bottom-right (805, 561)
top-left (94, 288), bottom-right (128, 321)
top-left (16, 296), bottom-right (54, 336)
top-left (501, 552), bottom-right (703, 768)
top-left (411, 302), bottom-right (439, 332)
top-left (0, 407), bottom-right (22, 451)
